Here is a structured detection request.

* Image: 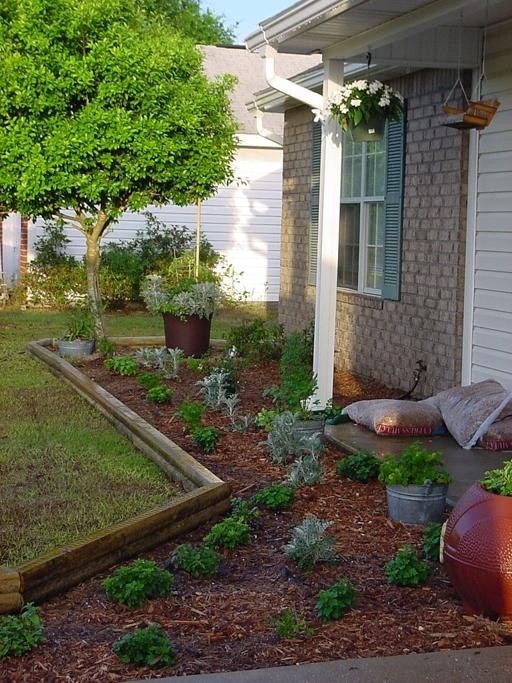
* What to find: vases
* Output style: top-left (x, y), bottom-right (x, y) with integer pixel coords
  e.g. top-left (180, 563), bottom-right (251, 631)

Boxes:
top-left (346, 115), bottom-right (385, 143)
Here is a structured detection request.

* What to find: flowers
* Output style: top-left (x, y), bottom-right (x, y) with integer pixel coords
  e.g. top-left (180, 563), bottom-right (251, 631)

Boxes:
top-left (312, 79), bottom-right (406, 130)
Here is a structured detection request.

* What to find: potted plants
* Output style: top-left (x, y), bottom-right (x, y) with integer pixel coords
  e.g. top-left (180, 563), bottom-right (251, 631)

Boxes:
top-left (379, 438), bottom-right (453, 525)
top-left (443, 456), bottom-right (511, 621)
top-left (56, 303), bottom-right (98, 357)
top-left (139, 250), bottom-right (228, 357)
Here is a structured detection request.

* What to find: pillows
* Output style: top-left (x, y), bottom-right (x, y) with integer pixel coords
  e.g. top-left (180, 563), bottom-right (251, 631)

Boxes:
top-left (341, 377), bottom-right (511, 453)
